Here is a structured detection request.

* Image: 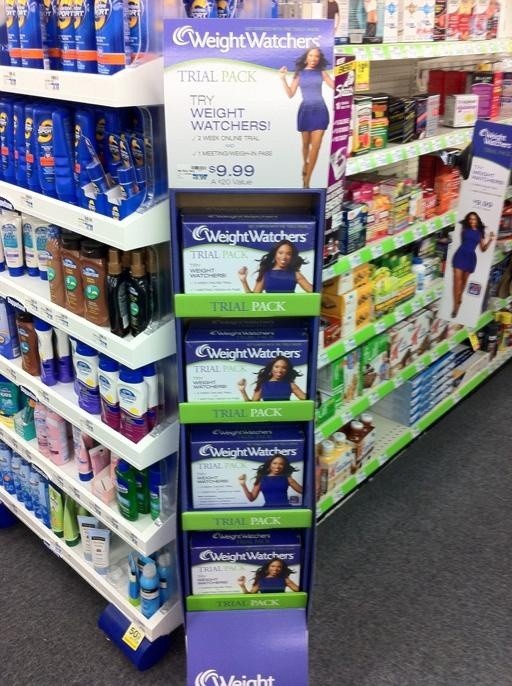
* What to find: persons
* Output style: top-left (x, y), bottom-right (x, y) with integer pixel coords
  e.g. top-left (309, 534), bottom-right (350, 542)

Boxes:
top-left (238, 355), bottom-right (307, 400)
top-left (280, 44), bottom-right (334, 189)
top-left (240, 454), bottom-right (301, 506)
top-left (452, 211), bottom-right (495, 318)
top-left (239, 239), bottom-right (313, 293)
top-left (238, 558), bottom-right (299, 593)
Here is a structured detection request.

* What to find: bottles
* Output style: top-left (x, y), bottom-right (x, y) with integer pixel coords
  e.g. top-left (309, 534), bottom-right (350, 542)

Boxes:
top-left (122, 541), bottom-right (180, 627)
top-left (114, 460), bottom-right (169, 523)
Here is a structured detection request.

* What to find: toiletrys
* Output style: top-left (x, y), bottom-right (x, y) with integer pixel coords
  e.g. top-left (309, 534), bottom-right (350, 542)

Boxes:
top-left (0, 0), bottom-right (242, 618)
